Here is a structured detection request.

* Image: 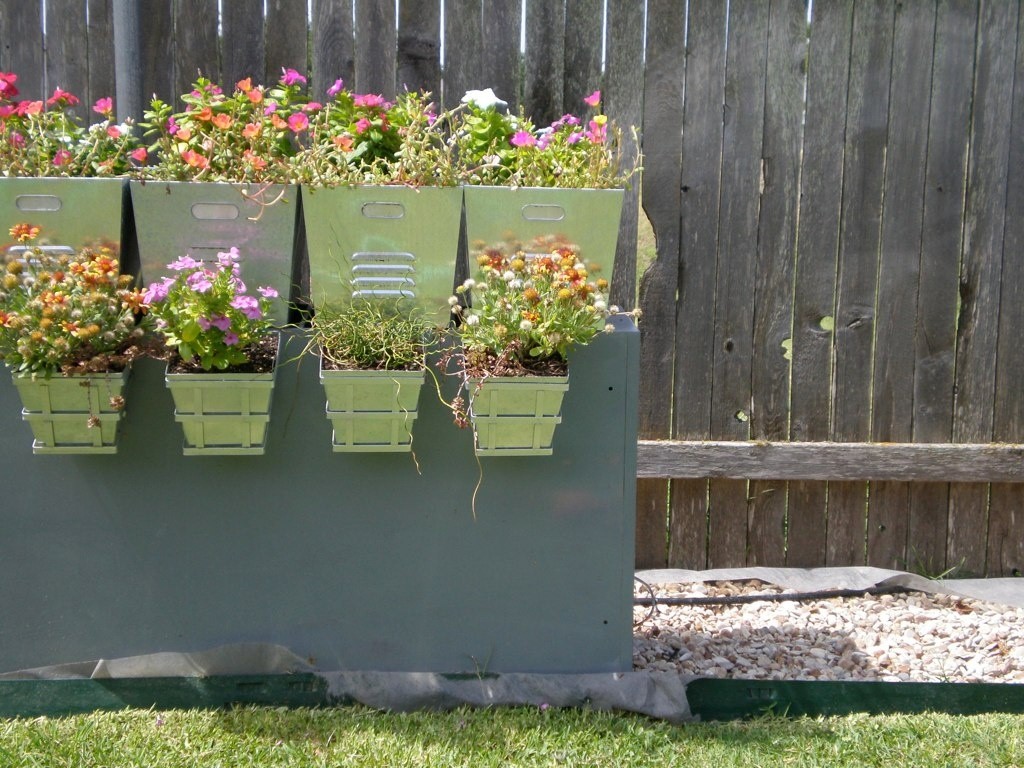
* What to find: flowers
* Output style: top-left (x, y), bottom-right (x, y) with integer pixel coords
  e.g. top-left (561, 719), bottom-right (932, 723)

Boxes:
top-left (0, 65), bottom-right (646, 221)
top-left (141, 246), bottom-right (280, 372)
top-left (0, 222), bottom-right (177, 429)
top-left (434, 227), bottom-right (645, 431)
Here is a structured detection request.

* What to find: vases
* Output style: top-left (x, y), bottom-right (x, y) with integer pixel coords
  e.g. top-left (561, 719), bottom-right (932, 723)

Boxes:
top-left (460, 342), bottom-right (571, 458)
top-left (0, 175), bottom-right (131, 276)
top-left (128, 179), bottom-right (302, 331)
top-left (301, 183), bottom-right (465, 330)
top-left (463, 185), bottom-right (626, 310)
top-left (162, 330), bottom-right (282, 457)
top-left (10, 360), bottom-right (133, 456)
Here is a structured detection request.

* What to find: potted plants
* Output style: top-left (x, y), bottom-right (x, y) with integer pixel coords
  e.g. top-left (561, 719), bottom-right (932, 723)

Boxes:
top-left (275, 233), bottom-right (483, 524)
